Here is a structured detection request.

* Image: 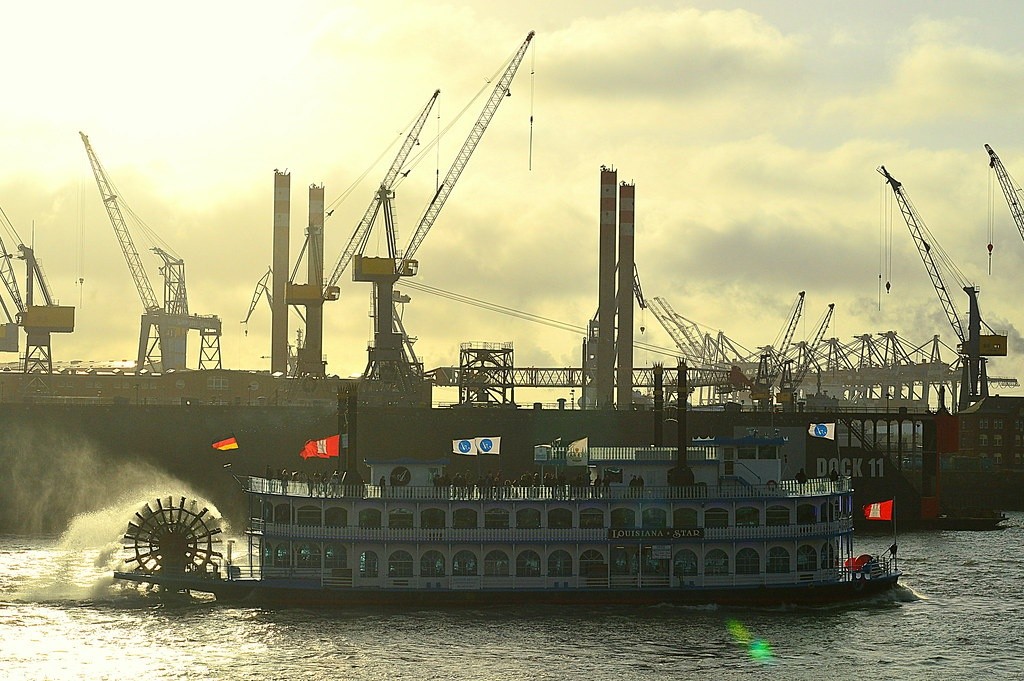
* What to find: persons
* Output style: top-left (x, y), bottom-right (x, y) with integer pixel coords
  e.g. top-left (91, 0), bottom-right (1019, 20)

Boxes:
top-left (795, 468), bottom-right (809, 493)
top-left (434, 469), bottom-right (611, 502)
top-left (278, 469), bottom-right (288, 497)
top-left (379, 476), bottom-right (386, 498)
top-left (306, 471), bottom-right (340, 498)
top-left (830, 467), bottom-right (839, 482)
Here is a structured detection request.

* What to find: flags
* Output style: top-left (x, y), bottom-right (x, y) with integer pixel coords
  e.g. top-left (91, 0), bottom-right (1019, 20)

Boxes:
top-left (807, 422), bottom-right (835, 441)
top-left (864, 501), bottom-right (893, 521)
top-left (452, 439), bottom-right (477, 457)
top-left (569, 437), bottom-right (588, 459)
top-left (475, 437), bottom-right (501, 455)
top-left (316, 434), bottom-right (339, 457)
top-left (212, 436), bottom-right (239, 451)
top-left (300, 441), bottom-right (329, 460)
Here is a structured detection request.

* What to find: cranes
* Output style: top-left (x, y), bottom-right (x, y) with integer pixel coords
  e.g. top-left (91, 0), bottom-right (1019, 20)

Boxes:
top-left (0, 202), bottom-right (75, 397)
top-left (283, 89), bottom-right (441, 404)
top-left (639, 288), bottom-right (836, 403)
top-left (874, 164), bottom-right (1007, 410)
top-left (78, 130), bottom-right (230, 372)
top-left (351, 30), bottom-right (536, 404)
top-left (985, 143), bottom-right (1024, 276)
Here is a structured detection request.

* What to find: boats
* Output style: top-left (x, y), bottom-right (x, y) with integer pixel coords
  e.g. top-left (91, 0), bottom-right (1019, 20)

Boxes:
top-left (939, 510), bottom-right (1010, 529)
top-left (115, 356), bottom-right (901, 608)
